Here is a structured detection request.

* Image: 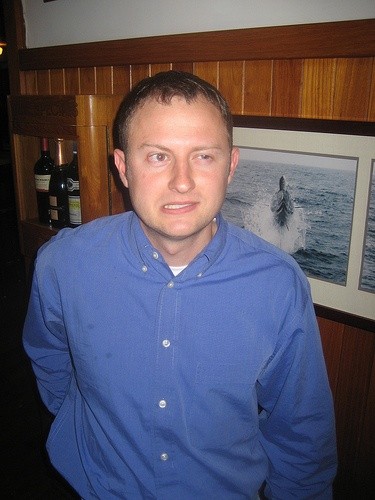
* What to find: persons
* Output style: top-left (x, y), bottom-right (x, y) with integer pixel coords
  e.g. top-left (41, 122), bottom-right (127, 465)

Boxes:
top-left (23, 70), bottom-right (338, 500)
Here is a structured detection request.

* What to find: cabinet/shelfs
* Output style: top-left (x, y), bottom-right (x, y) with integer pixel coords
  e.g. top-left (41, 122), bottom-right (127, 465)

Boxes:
top-left (5, 93), bottom-right (127, 292)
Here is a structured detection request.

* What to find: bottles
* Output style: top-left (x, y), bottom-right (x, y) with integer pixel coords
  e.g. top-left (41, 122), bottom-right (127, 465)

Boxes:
top-left (34, 138), bottom-right (55, 223)
top-left (50, 138), bottom-right (69, 229)
top-left (66, 141), bottom-right (82, 228)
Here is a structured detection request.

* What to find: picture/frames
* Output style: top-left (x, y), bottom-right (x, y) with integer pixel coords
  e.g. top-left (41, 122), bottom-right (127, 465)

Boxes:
top-left (217, 114), bottom-right (375, 329)
